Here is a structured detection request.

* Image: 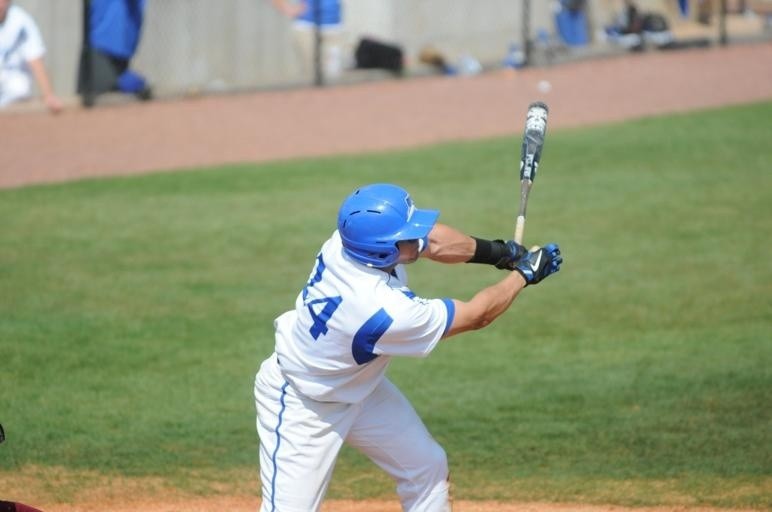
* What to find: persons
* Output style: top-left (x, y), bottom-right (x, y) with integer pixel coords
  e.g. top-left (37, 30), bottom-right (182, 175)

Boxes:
top-left (538, 0), bottom-right (675, 50)
top-left (0, 424), bottom-right (42, 512)
top-left (276, 0), bottom-right (343, 85)
top-left (0, 0), bottom-right (59, 115)
top-left (254, 183), bottom-right (563, 512)
top-left (77, 0), bottom-right (150, 106)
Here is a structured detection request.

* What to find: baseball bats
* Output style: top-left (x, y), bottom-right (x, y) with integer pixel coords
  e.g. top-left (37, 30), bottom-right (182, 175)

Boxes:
top-left (508, 101), bottom-right (549, 270)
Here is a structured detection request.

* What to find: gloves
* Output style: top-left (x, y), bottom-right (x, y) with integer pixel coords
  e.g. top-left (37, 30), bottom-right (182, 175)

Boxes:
top-left (488, 239), bottom-right (564, 287)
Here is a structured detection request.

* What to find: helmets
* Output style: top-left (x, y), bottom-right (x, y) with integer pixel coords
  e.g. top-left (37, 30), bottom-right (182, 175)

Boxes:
top-left (337, 183), bottom-right (440, 268)
